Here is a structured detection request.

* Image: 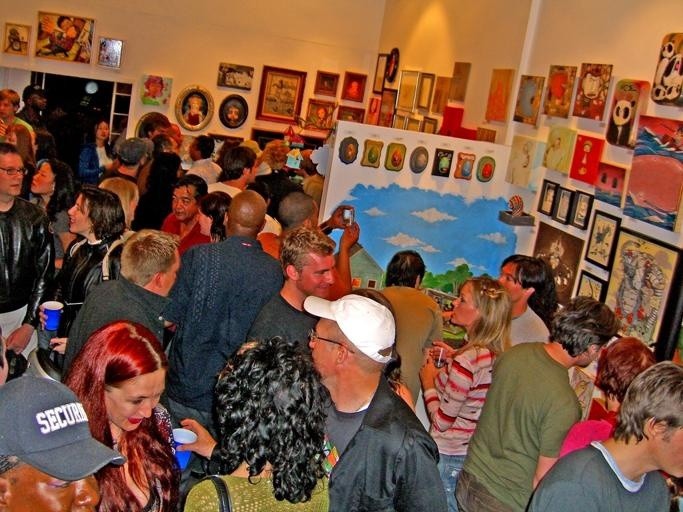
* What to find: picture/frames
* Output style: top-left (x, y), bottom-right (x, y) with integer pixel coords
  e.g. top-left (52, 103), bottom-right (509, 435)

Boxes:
top-left (217, 61), bottom-right (255, 92)
top-left (2, 23), bottom-right (32, 57)
top-left (537, 179), bottom-right (560, 216)
top-left (576, 271), bottom-right (607, 303)
top-left (364, 48), bottom-right (438, 162)
top-left (251, 128), bottom-right (323, 152)
top-left (664, 285), bottom-right (683, 368)
top-left (305, 98), bottom-right (338, 134)
top-left (254, 63), bottom-right (309, 126)
top-left (171, 83), bottom-right (215, 131)
top-left (551, 186), bottom-right (576, 225)
top-left (139, 73), bottom-right (174, 110)
top-left (313, 69), bottom-right (341, 97)
top-left (35, 11), bottom-right (96, 66)
top-left (603, 227), bottom-right (683, 362)
top-left (570, 190), bottom-right (594, 231)
top-left (337, 104), bottom-right (366, 125)
top-left (218, 93), bottom-right (249, 130)
top-left (584, 209), bottom-right (622, 271)
top-left (341, 70), bottom-right (368, 102)
top-left (97, 36), bottom-right (126, 72)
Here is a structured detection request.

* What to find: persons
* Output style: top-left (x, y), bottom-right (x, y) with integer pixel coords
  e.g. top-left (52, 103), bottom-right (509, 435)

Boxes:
top-left (1, 84), bottom-right (682, 512)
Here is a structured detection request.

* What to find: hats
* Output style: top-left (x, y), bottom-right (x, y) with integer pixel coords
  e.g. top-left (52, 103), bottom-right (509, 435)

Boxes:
top-left (303, 294), bottom-right (396, 364)
top-left (0, 376), bottom-right (127, 480)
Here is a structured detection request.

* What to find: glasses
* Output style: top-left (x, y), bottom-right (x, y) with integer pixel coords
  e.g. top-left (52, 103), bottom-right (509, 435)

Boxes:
top-left (1, 168), bottom-right (25, 176)
top-left (306, 328), bottom-right (356, 356)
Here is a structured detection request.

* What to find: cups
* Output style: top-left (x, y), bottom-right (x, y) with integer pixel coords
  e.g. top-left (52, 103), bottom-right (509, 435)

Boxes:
top-left (431, 347), bottom-right (447, 370)
top-left (40, 300), bottom-right (64, 332)
top-left (172, 428), bottom-right (197, 471)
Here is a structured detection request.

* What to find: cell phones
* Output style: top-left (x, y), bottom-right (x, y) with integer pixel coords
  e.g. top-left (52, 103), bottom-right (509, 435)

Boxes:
top-left (341, 208), bottom-right (355, 226)
